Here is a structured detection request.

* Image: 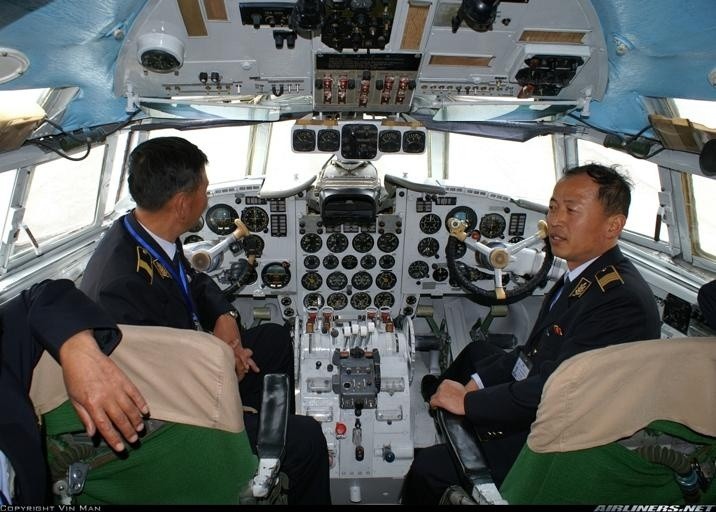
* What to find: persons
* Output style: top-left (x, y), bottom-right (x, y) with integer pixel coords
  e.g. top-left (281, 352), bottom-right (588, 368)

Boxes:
top-left (398, 164), bottom-right (661, 507)
top-left (0, 279), bottom-right (149, 505)
top-left (80, 137), bottom-right (331, 505)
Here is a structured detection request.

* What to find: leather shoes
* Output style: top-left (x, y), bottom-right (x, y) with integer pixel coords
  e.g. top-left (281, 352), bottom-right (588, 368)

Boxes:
top-left (421, 374), bottom-right (439, 418)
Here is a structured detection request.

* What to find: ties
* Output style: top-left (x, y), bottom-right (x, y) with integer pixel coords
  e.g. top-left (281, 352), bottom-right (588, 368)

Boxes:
top-left (549, 276), bottom-right (570, 310)
top-left (173, 252), bottom-right (182, 280)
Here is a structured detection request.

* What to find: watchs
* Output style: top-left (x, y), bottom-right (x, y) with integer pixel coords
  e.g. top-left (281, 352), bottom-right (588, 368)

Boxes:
top-left (229, 310), bottom-right (238, 318)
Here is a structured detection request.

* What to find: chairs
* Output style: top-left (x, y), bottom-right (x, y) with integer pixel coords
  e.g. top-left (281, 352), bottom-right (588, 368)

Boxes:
top-left (28, 322), bottom-right (293, 505)
top-left (432, 332), bottom-right (714, 505)
